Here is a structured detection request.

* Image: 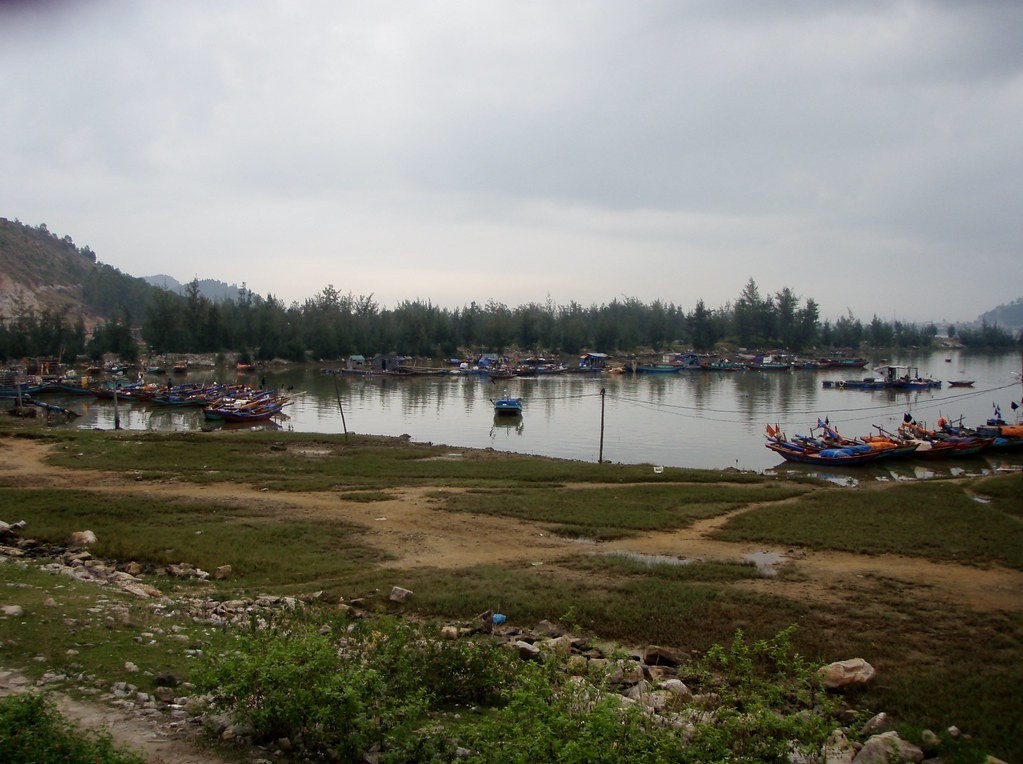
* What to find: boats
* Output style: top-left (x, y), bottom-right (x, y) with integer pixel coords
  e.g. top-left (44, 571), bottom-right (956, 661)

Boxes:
top-left (822, 366), bottom-right (942, 389)
top-left (382, 353), bottom-right (613, 379)
top-left (623, 352), bottom-right (869, 374)
top-left (765, 419), bottom-right (1023, 468)
top-left (947, 380), bottom-right (975, 386)
top-left (494, 399), bottom-right (523, 415)
top-left (0, 361), bottom-right (307, 424)
